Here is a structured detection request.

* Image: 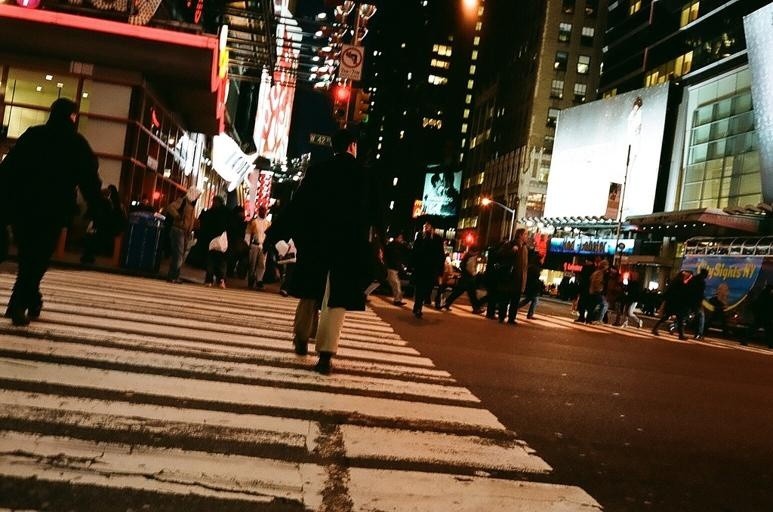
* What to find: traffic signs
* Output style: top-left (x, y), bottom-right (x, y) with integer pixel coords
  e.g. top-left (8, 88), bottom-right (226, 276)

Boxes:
top-left (309, 133), bottom-right (333, 149)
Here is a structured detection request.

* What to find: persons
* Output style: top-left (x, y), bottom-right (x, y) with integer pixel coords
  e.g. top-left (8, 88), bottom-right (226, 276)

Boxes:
top-left (81, 185), bottom-right (287, 297)
top-left (263, 129), bottom-right (387, 376)
top-left (363, 221), bottom-right (543, 324)
top-left (1, 99), bottom-right (102, 325)
top-left (571, 260), bottom-right (731, 342)
top-left (424, 172), bottom-right (459, 214)
top-left (628, 97), bottom-right (644, 165)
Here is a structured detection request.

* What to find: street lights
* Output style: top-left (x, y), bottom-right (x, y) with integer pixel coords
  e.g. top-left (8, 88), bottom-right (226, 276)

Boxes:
top-left (481, 195), bottom-right (516, 242)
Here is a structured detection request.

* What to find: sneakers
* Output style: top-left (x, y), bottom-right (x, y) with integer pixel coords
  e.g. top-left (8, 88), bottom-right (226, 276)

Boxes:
top-left (620, 319), bottom-right (689, 340)
top-left (394, 300), bottom-right (518, 325)
top-left (294, 337), bottom-right (308, 355)
top-left (5, 310), bottom-right (30, 326)
top-left (318, 360), bottom-right (333, 374)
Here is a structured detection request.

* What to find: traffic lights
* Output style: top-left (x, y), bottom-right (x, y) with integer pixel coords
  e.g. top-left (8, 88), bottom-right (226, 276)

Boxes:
top-left (353, 87), bottom-right (374, 123)
top-left (331, 86), bottom-right (350, 126)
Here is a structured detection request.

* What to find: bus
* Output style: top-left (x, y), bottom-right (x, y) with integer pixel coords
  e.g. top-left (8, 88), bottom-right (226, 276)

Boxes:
top-left (679, 237), bottom-right (772, 345)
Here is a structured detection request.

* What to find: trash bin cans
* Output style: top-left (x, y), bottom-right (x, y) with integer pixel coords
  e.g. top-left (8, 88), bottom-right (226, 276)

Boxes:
top-left (118, 205), bottom-right (168, 279)
